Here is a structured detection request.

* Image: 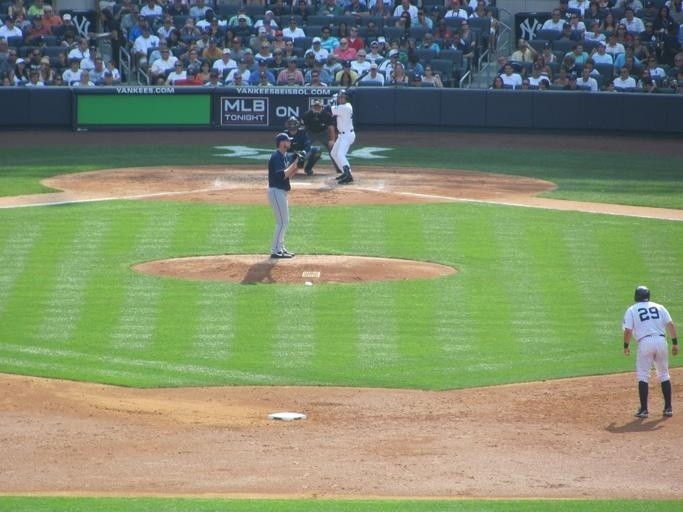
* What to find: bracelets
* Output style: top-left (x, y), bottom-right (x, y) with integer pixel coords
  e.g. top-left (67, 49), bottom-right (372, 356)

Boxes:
top-left (624, 343), bottom-right (628, 348)
top-left (672, 338), bottom-right (677, 345)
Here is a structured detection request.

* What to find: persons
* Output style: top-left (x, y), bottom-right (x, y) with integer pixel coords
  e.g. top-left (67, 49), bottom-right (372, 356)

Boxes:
top-left (1, 0), bottom-right (682, 96)
top-left (283, 116), bottom-right (321, 175)
top-left (303, 98), bottom-right (342, 173)
top-left (623, 286), bottom-right (679, 417)
top-left (267, 133), bottom-right (299, 258)
top-left (322, 89), bottom-right (355, 183)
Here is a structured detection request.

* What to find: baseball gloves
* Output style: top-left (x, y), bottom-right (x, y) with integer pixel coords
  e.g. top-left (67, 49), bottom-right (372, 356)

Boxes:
top-left (293, 152), bottom-right (304, 169)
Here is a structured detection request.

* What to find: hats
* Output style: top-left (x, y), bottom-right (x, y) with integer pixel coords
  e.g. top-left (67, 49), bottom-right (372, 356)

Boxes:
top-left (275, 132), bottom-right (294, 141)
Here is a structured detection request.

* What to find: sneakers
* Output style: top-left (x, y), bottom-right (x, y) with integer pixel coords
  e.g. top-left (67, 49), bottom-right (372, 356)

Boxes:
top-left (303, 166), bottom-right (315, 177)
top-left (634, 408), bottom-right (648, 418)
top-left (663, 408), bottom-right (673, 417)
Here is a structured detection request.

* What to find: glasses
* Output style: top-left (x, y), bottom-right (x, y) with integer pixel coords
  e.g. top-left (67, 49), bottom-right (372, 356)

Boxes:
top-left (133, 7), bottom-right (466, 86)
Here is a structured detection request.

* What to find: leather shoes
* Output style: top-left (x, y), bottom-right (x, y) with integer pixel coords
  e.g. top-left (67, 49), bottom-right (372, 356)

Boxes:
top-left (270, 249), bottom-right (295, 258)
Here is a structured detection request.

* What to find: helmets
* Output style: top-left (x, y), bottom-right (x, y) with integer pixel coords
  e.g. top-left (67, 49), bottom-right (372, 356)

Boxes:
top-left (634, 286), bottom-right (650, 302)
top-left (285, 116), bottom-right (300, 131)
top-left (312, 98), bottom-right (324, 113)
top-left (338, 89), bottom-right (350, 99)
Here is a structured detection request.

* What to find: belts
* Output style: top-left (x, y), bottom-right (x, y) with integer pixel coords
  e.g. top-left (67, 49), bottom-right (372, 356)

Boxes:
top-left (337, 129), bottom-right (354, 134)
top-left (639, 334), bottom-right (665, 342)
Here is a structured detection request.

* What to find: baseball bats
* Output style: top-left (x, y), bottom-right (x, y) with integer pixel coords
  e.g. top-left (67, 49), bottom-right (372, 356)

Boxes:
top-left (344, 69), bottom-right (369, 91)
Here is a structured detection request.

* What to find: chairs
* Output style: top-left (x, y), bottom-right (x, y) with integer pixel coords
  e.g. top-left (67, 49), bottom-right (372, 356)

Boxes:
top-left (2, 7), bottom-right (678, 89)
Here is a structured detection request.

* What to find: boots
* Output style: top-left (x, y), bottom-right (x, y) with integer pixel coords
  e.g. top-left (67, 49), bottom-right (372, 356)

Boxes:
top-left (335, 166), bottom-right (353, 184)
